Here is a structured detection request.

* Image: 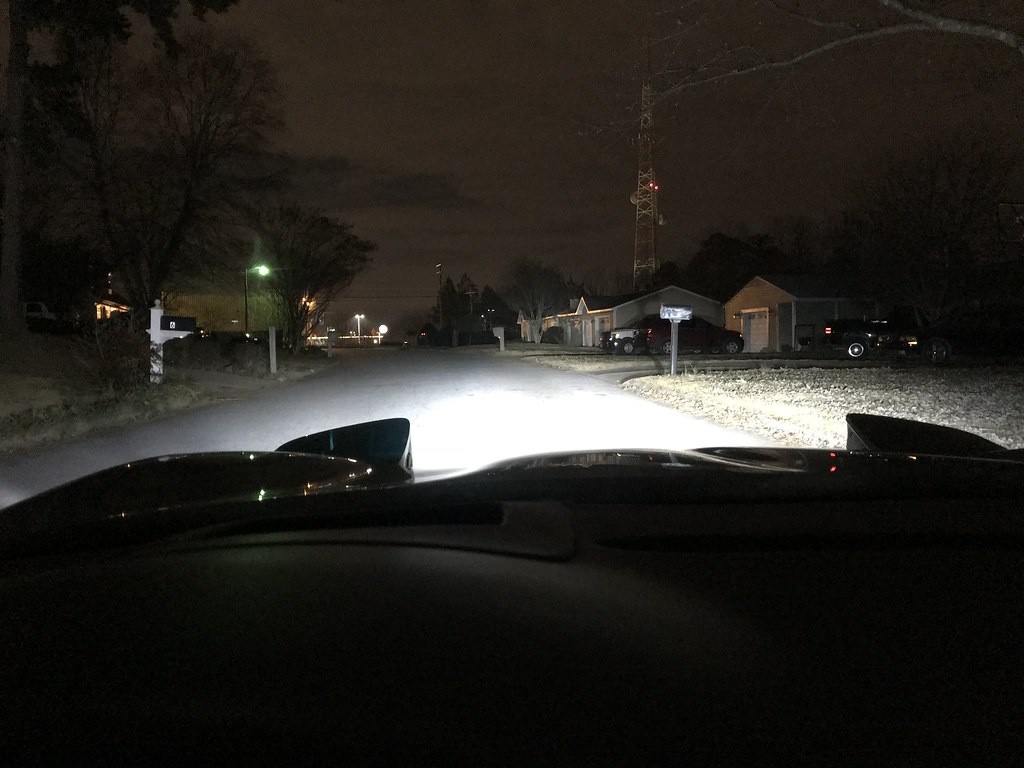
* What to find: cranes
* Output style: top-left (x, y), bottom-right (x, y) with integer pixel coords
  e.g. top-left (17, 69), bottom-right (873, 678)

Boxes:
top-left (632, 0), bottom-right (668, 301)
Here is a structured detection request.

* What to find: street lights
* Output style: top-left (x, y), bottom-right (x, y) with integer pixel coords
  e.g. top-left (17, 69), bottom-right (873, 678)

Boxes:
top-left (353, 311), bottom-right (365, 347)
top-left (247, 261), bottom-right (267, 339)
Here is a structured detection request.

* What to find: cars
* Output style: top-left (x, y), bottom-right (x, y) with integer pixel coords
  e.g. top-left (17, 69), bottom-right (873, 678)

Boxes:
top-left (633, 311), bottom-right (745, 357)
top-left (602, 307), bottom-right (642, 356)
top-left (822, 297), bottom-right (1023, 370)
